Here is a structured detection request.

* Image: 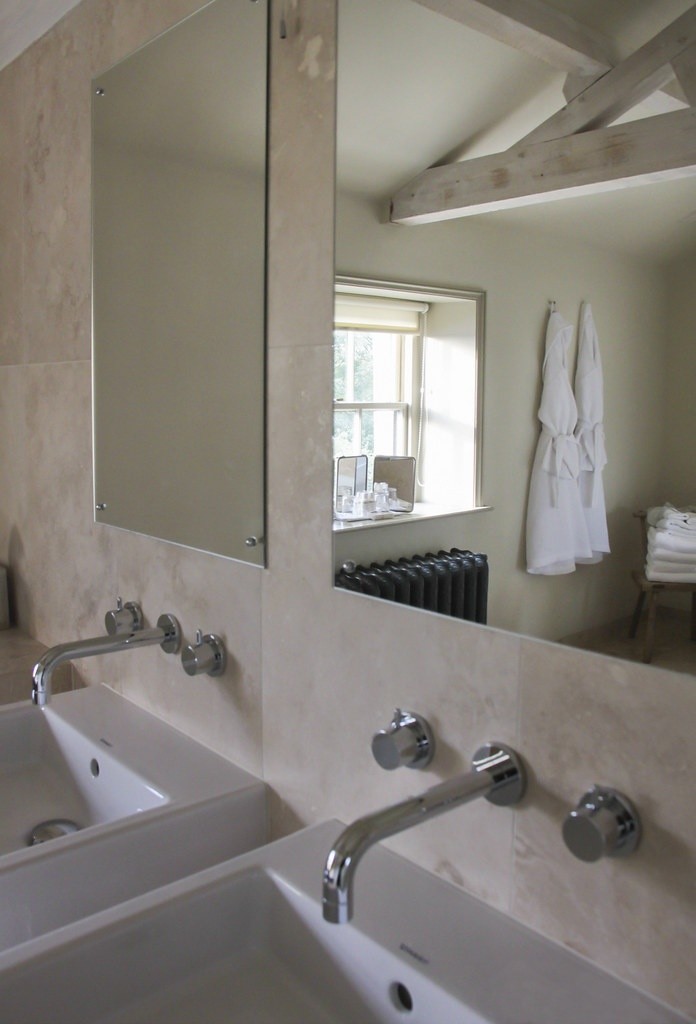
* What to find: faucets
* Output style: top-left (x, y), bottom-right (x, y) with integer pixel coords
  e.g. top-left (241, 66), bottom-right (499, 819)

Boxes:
top-left (320, 740), bottom-right (530, 927)
top-left (30, 612), bottom-right (182, 708)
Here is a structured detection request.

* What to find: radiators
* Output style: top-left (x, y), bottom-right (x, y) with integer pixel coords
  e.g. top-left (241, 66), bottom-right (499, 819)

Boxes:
top-left (335, 546), bottom-right (487, 625)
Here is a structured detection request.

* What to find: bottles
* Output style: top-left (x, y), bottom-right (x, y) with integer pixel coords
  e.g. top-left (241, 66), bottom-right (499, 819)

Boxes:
top-left (343, 482), bottom-right (397, 518)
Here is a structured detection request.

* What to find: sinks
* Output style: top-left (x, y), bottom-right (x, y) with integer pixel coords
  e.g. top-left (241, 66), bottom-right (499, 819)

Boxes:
top-left (1, 818), bottom-right (694, 1024)
top-left (1, 680), bottom-right (269, 952)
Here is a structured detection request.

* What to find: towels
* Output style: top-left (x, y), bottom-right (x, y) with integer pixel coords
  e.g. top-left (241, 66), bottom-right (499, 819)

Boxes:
top-left (644, 500), bottom-right (696, 584)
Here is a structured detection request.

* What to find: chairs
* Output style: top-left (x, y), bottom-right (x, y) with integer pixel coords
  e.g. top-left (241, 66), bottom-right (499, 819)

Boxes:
top-left (629, 505), bottom-right (696, 665)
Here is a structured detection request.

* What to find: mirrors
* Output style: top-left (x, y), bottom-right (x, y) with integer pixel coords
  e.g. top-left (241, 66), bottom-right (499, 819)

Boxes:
top-left (324, 0), bottom-right (696, 681)
top-left (371, 452), bottom-right (417, 514)
top-left (333, 453), bottom-right (368, 517)
top-left (88, 0), bottom-right (269, 572)
